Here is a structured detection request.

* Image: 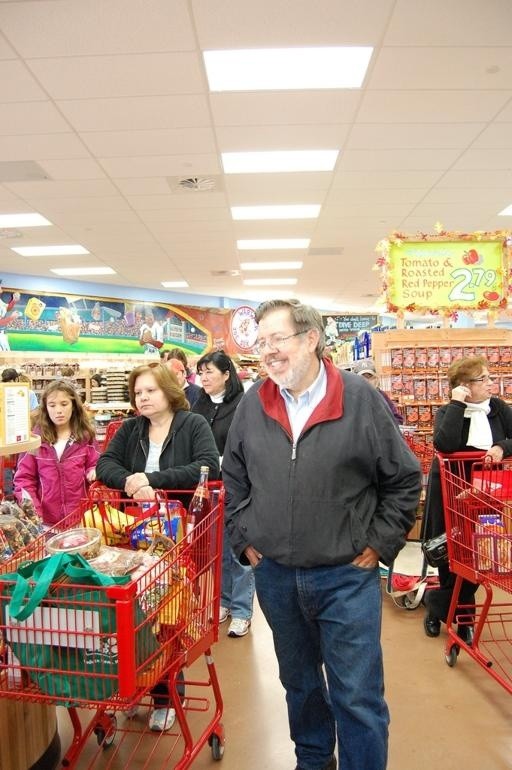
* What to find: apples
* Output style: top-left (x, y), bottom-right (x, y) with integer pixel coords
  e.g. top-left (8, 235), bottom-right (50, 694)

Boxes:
top-left (463, 249), bottom-right (478, 265)
top-left (483, 291), bottom-right (498, 301)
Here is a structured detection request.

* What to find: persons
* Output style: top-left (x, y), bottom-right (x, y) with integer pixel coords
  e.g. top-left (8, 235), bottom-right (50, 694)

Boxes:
top-left (0, 346), bottom-right (256, 731)
top-left (355, 360), bottom-right (402, 426)
top-left (420, 356), bottom-right (511, 650)
top-left (222, 299), bottom-right (421, 769)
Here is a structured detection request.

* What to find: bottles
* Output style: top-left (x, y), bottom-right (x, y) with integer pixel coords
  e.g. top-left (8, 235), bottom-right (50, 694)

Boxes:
top-left (184, 465), bottom-right (211, 575)
top-left (354, 324), bottom-right (397, 360)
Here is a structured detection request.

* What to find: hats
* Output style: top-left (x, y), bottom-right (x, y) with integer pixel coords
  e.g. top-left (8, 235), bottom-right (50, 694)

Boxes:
top-left (353, 358), bottom-right (378, 378)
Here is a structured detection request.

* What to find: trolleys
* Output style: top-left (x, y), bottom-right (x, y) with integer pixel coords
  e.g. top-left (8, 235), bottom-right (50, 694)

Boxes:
top-left (431, 450), bottom-right (511, 693)
top-left (0, 477), bottom-right (228, 770)
top-left (384, 430), bottom-right (442, 611)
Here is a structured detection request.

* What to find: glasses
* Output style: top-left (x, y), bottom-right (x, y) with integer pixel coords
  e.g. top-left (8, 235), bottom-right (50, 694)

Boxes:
top-left (469, 375), bottom-right (492, 385)
top-left (251, 329), bottom-right (307, 356)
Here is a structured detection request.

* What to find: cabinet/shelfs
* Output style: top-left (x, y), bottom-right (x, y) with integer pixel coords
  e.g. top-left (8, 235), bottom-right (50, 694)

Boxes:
top-left (1, 433), bottom-right (63, 769)
top-left (337, 329), bottom-right (512, 541)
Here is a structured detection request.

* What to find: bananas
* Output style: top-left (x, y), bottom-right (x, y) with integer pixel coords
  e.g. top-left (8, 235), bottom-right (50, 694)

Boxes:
top-left (79, 501), bottom-right (145, 547)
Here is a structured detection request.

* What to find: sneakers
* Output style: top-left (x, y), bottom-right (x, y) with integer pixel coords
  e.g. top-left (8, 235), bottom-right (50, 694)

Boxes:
top-left (120, 693), bottom-right (142, 719)
top-left (148, 698), bottom-right (187, 732)
top-left (207, 603), bottom-right (231, 624)
top-left (226, 615), bottom-right (252, 637)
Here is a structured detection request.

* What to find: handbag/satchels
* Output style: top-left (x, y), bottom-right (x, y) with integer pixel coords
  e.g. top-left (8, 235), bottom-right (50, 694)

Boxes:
top-left (0, 549), bottom-right (166, 709)
top-left (420, 524), bottom-right (469, 569)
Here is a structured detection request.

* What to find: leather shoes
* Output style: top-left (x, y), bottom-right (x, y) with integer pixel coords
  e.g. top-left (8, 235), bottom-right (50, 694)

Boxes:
top-left (456, 623), bottom-right (475, 647)
top-left (424, 589), bottom-right (441, 637)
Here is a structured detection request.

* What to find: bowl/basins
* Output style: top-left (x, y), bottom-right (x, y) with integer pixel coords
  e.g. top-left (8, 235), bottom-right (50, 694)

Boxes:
top-left (45, 527), bottom-right (103, 563)
